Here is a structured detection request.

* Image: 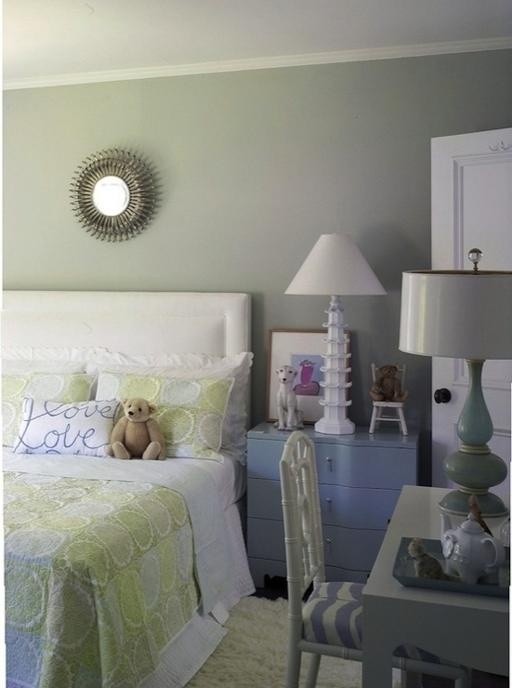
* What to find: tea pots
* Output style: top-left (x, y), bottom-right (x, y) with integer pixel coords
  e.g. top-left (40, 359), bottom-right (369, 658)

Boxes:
top-left (439, 512), bottom-right (506, 586)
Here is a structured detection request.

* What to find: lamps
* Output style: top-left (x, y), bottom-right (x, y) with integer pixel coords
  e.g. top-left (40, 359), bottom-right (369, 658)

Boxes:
top-left (286, 233), bottom-right (389, 436)
top-left (399, 248), bottom-right (512, 518)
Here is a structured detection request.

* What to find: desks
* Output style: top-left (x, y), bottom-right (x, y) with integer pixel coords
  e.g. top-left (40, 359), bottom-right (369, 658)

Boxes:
top-left (360, 485), bottom-right (508, 683)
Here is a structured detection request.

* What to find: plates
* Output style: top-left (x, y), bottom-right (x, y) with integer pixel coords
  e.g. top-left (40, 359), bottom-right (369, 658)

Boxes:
top-left (392, 536), bottom-right (509, 598)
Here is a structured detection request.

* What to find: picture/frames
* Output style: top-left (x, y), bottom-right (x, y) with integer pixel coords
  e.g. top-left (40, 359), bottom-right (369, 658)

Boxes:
top-left (265, 328), bottom-right (350, 425)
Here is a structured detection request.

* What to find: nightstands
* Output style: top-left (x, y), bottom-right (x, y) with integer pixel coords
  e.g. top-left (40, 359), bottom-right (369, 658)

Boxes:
top-left (246, 423), bottom-right (424, 591)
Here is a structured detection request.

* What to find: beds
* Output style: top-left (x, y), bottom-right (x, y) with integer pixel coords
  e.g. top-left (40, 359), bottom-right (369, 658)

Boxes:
top-left (3, 291), bottom-right (254, 683)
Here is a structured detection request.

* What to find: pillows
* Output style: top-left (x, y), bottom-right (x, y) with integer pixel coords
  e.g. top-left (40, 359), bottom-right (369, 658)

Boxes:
top-left (4, 371), bottom-right (98, 448)
top-left (95, 370), bottom-right (234, 461)
top-left (91, 346), bottom-right (254, 466)
top-left (2, 349), bottom-right (107, 374)
top-left (10, 396), bottom-right (125, 459)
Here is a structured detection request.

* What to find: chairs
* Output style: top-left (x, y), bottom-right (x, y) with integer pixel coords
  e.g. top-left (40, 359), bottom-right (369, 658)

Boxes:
top-left (279, 430), bottom-right (472, 683)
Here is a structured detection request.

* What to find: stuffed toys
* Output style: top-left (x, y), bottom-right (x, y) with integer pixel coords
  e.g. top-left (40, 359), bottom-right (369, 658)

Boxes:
top-left (108, 396), bottom-right (166, 460)
top-left (370, 365), bottom-right (407, 404)
top-left (274, 364), bottom-right (303, 431)
